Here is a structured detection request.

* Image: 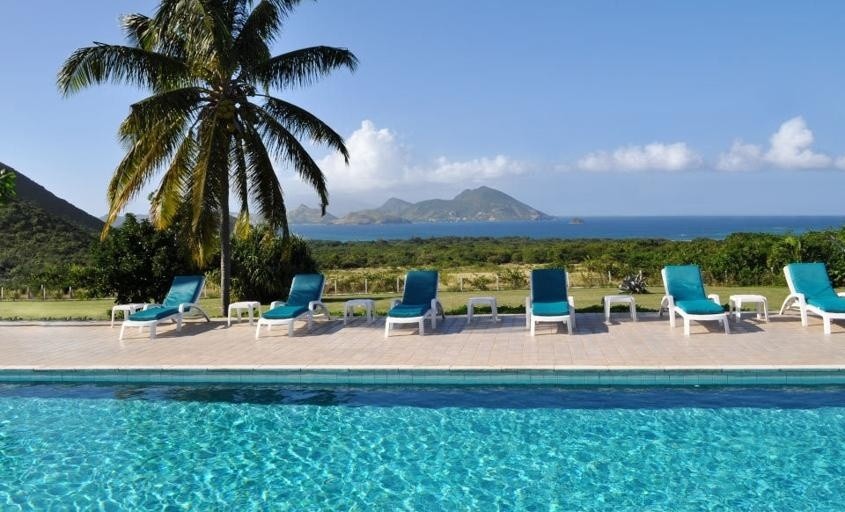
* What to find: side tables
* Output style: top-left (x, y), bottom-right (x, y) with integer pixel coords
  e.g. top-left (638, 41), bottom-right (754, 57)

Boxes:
top-left (467, 297), bottom-right (499, 325)
top-left (342, 298), bottom-right (375, 326)
top-left (225, 299), bottom-right (261, 329)
top-left (603, 295), bottom-right (637, 323)
top-left (730, 295), bottom-right (770, 324)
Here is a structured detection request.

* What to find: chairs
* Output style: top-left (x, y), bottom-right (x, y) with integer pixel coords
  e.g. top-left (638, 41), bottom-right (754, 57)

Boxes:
top-left (657, 264), bottom-right (731, 335)
top-left (525, 269), bottom-right (576, 337)
top-left (254, 273), bottom-right (332, 337)
top-left (384, 271), bottom-right (446, 337)
top-left (777, 262), bottom-right (845, 335)
top-left (111, 274), bottom-right (211, 337)
